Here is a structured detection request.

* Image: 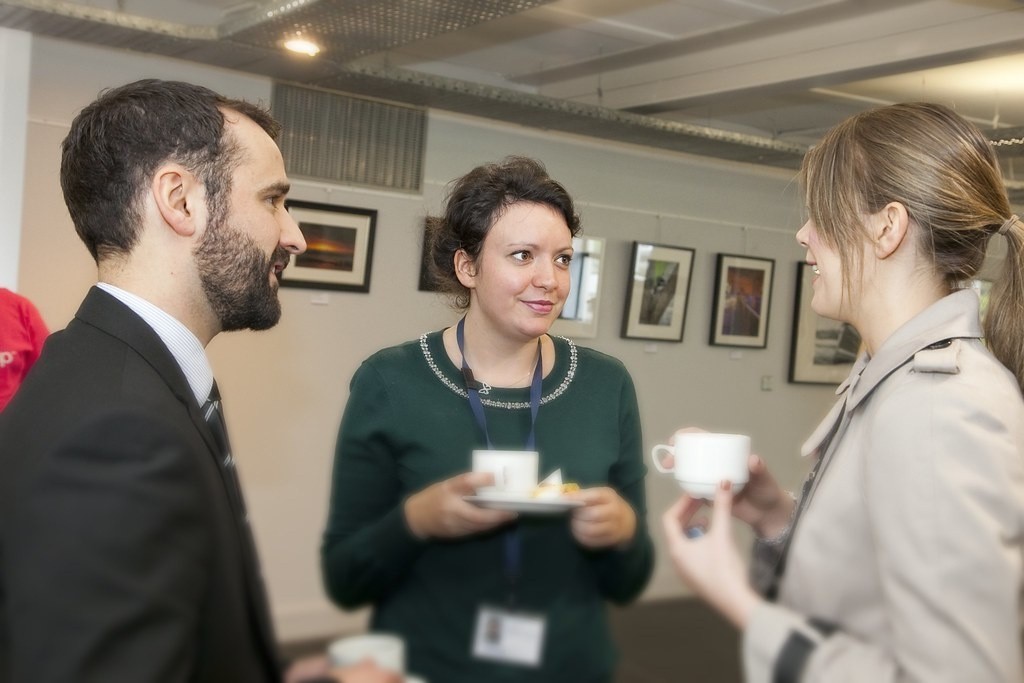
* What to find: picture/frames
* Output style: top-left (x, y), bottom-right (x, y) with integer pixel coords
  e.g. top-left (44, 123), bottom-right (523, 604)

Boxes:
top-left (707, 251), bottom-right (776, 349)
top-left (277, 197), bottom-right (379, 295)
top-left (787, 261), bottom-right (853, 386)
top-left (620, 239), bottom-right (696, 344)
top-left (550, 236), bottom-right (605, 339)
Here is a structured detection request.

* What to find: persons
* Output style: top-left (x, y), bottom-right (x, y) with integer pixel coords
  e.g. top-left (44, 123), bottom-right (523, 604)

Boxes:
top-left (664, 102), bottom-right (1022, 683)
top-left (319, 156), bottom-right (656, 683)
top-left (0, 78), bottom-right (404, 682)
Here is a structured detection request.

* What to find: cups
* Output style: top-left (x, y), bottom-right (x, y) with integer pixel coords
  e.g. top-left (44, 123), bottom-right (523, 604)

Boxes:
top-left (471, 450), bottom-right (538, 497)
top-left (652, 434), bottom-right (751, 501)
top-left (330, 634), bottom-right (405, 672)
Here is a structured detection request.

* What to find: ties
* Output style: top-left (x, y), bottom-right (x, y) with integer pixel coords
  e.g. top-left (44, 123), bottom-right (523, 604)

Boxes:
top-left (205, 379), bottom-right (231, 463)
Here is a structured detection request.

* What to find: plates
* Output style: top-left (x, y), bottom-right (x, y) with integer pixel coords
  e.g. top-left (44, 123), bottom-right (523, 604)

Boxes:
top-left (405, 673), bottom-right (424, 683)
top-left (463, 497), bottom-right (584, 514)
